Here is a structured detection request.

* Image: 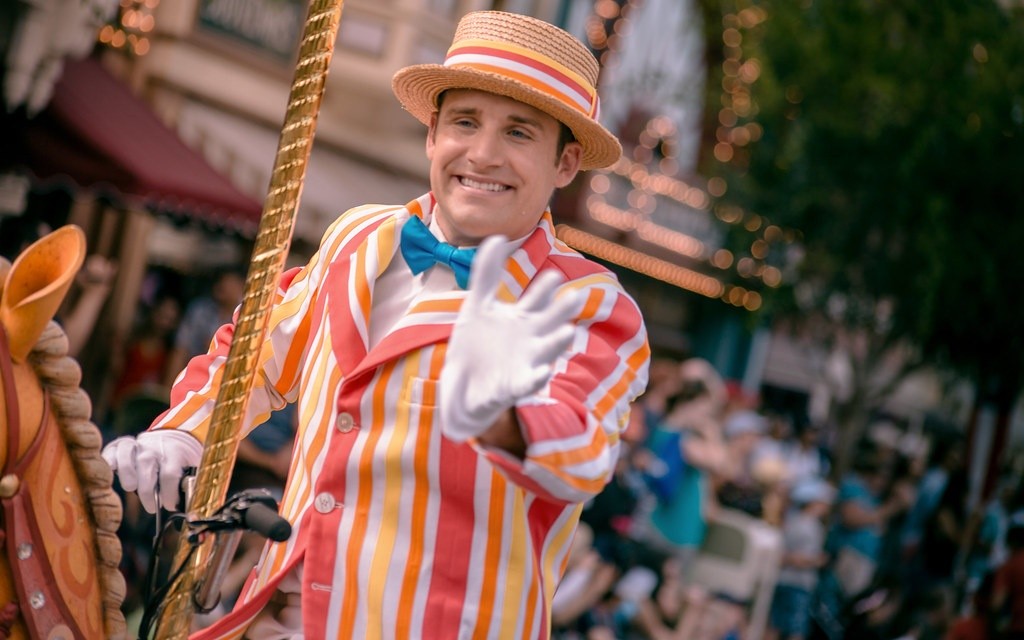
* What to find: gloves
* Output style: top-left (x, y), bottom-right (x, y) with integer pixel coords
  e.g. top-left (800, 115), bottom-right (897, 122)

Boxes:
top-left (439, 233), bottom-right (584, 444)
top-left (101, 429), bottom-right (204, 515)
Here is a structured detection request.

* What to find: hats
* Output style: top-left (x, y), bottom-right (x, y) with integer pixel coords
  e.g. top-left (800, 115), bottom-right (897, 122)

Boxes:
top-left (391, 9), bottom-right (622, 171)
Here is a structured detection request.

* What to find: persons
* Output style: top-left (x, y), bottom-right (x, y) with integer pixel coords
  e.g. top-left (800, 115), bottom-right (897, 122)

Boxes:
top-left (99, 11), bottom-right (652, 640)
top-left (1, 211), bottom-right (1024, 640)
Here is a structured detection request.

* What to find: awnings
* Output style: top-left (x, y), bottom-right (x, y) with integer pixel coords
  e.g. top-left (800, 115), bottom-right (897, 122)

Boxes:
top-left (46, 54), bottom-right (264, 237)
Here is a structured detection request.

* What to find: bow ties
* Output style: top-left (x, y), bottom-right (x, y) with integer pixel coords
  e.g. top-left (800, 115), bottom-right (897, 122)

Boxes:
top-left (400, 215), bottom-right (477, 291)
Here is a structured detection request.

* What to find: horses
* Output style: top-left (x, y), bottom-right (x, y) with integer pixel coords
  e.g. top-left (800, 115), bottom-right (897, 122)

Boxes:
top-left (0, 224), bottom-right (133, 640)
